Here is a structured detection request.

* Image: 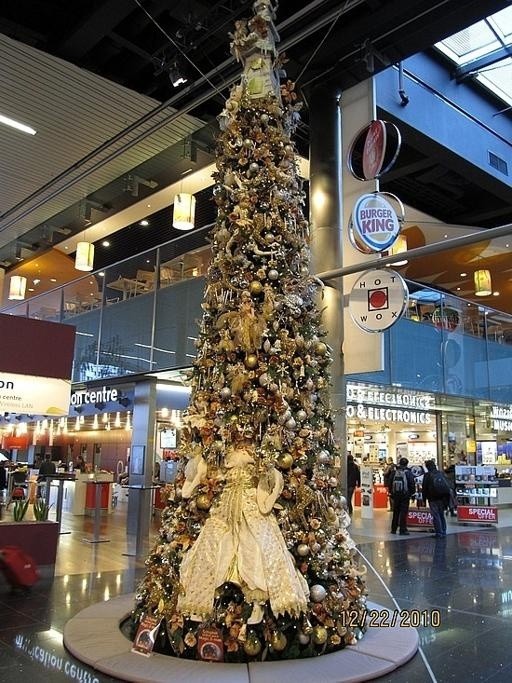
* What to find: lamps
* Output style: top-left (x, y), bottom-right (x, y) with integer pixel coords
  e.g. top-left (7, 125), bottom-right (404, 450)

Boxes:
top-left (173, 135), bottom-right (196, 231)
top-left (388, 233), bottom-right (408, 266)
top-left (75, 224), bottom-right (95, 272)
top-left (167, 65), bottom-right (187, 88)
top-left (8, 275), bottom-right (27, 301)
top-left (475, 255), bottom-right (493, 297)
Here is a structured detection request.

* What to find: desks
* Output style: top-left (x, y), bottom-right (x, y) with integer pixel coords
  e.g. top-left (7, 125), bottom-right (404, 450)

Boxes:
top-left (160, 243), bottom-right (212, 275)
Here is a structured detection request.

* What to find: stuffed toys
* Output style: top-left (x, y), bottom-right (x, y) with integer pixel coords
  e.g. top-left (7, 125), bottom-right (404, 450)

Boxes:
top-left (176, 434), bottom-right (311, 624)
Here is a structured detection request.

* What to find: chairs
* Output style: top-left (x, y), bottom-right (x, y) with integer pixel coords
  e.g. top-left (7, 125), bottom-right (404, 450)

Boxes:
top-left (20, 254), bottom-right (203, 322)
top-left (404, 299), bottom-right (512, 345)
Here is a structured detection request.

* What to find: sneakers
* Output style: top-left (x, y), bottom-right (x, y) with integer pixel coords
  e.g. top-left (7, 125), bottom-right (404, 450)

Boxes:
top-left (390, 529), bottom-right (410, 536)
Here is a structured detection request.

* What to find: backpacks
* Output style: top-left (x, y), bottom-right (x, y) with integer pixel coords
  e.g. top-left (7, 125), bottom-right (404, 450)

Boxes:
top-left (392, 468), bottom-right (410, 495)
top-left (429, 472), bottom-right (449, 499)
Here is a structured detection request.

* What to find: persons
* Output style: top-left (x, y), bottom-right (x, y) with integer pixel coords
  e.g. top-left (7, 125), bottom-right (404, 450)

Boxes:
top-left (382, 455), bottom-right (397, 512)
top-left (39, 453), bottom-right (56, 474)
top-left (443, 464), bottom-right (456, 517)
top-left (74, 455), bottom-right (86, 471)
top-left (387, 457), bottom-right (416, 535)
top-left (420, 460), bottom-right (447, 538)
top-left (33, 453), bottom-right (43, 466)
top-left (346, 454), bottom-right (361, 514)
